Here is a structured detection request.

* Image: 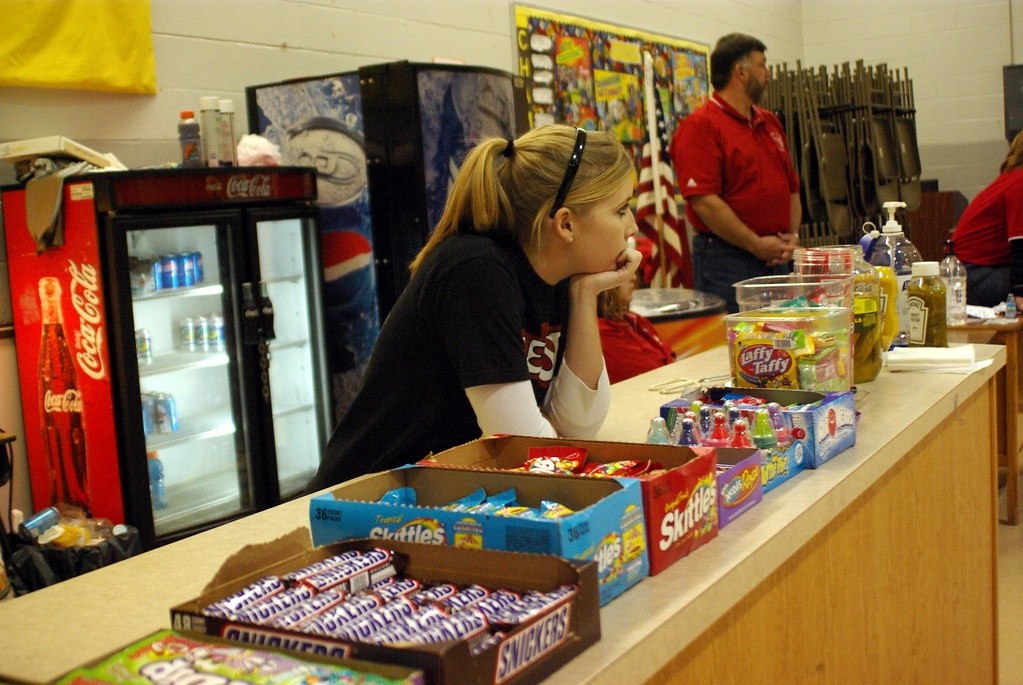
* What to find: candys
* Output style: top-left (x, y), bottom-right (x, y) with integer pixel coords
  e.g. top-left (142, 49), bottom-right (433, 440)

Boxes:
top-left (197, 320), bottom-right (853, 662)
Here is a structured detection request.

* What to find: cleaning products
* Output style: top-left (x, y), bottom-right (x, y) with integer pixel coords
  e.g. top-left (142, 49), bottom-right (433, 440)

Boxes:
top-left (940, 239), bottom-right (968, 328)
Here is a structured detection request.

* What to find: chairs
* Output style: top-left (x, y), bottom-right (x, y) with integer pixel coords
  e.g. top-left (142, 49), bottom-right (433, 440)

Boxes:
top-left (758, 56), bottom-right (924, 248)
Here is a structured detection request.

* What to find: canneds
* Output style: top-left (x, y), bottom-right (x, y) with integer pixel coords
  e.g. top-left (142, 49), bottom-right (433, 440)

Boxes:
top-left (178, 316), bottom-right (226, 353)
top-left (18, 506), bottom-right (61, 540)
top-left (141, 391), bottom-right (179, 437)
top-left (134, 328), bottom-right (152, 365)
top-left (152, 251), bottom-right (204, 290)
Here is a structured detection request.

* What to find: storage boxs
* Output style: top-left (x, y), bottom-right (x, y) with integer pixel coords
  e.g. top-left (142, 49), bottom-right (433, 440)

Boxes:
top-left (659, 386), bottom-right (857, 470)
top-left (733, 274), bottom-right (854, 373)
top-left (723, 307), bottom-right (857, 398)
top-left (41, 628), bottom-right (422, 685)
top-left (416, 433), bottom-right (720, 575)
top-left (673, 444), bottom-right (763, 531)
top-left (163, 528), bottom-right (600, 685)
top-left (302, 462), bottom-right (651, 606)
top-left (752, 442), bottom-right (804, 488)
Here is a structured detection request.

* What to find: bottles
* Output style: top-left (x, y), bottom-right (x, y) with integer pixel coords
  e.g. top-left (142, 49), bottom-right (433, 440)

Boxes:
top-left (145, 451), bottom-right (168, 511)
top-left (37, 277), bottom-right (91, 522)
top-left (1005, 294), bottom-right (1017, 318)
top-left (201, 95), bottom-right (224, 167)
top-left (217, 100), bottom-right (239, 166)
top-left (178, 110), bottom-right (201, 167)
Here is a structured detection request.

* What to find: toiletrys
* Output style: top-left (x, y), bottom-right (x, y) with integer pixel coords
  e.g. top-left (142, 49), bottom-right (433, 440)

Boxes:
top-left (869, 201), bottom-right (924, 346)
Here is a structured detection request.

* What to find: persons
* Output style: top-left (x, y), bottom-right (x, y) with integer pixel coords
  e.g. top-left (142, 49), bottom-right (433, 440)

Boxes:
top-left (301, 125), bottom-right (642, 494)
top-left (596, 275), bottom-right (676, 384)
top-left (672, 33), bottom-right (800, 314)
top-left (950, 130), bottom-right (1023, 312)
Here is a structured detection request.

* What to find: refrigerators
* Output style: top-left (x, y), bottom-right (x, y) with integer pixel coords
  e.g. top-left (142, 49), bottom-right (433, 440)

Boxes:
top-left (1, 166), bottom-right (336, 548)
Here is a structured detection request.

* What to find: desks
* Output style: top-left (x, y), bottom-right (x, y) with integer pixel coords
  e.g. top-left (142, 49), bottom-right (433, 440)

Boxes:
top-left (947, 315), bottom-right (1023, 527)
